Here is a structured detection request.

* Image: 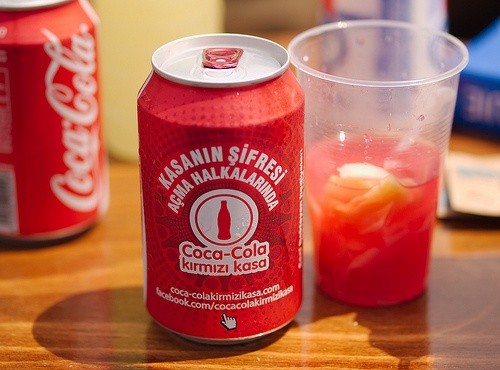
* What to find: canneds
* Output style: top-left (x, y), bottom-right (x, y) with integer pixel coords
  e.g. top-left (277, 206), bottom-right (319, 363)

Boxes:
top-left (137, 34), bottom-right (305, 342)
top-left (0, 0), bottom-right (113, 243)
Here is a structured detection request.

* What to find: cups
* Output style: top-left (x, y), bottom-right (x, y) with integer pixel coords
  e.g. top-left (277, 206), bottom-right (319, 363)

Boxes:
top-left (287, 18), bottom-right (469, 308)
